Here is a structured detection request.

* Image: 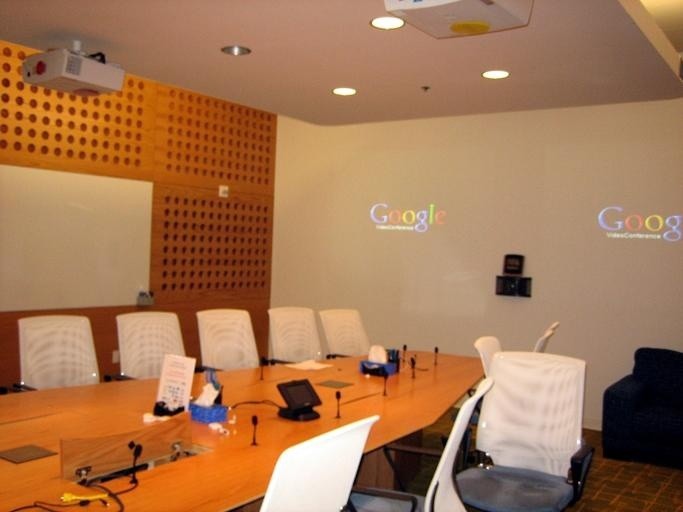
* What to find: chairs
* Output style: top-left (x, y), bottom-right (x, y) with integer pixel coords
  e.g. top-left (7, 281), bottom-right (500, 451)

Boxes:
top-left (261, 307), bottom-right (324, 366)
top-left (256, 413), bottom-right (381, 508)
top-left (1, 313), bottom-right (102, 397)
top-left (192, 308), bottom-right (261, 373)
top-left (318, 309), bottom-right (371, 360)
top-left (103, 311), bottom-right (188, 381)
top-left (600, 346), bottom-right (681, 469)
top-left (423, 319), bottom-right (596, 509)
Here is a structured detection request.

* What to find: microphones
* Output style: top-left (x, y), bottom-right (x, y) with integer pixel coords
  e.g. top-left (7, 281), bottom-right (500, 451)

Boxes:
top-left (335, 391), bottom-right (341, 418)
top-left (130, 444), bottom-right (142, 483)
top-left (434, 347), bottom-right (438, 365)
top-left (383, 374), bottom-right (388, 396)
top-left (403, 345), bottom-right (407, 363)
top-left (251, 416), bottom-right (258, 446)
top-left (260, 357), bottom-right (265, 380)
top-left (411, 358), bottom-right (415, 378)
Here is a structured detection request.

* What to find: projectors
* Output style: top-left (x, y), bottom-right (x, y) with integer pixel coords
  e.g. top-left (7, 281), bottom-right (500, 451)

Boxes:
top-left (22, 51), bottom-right (125, 96)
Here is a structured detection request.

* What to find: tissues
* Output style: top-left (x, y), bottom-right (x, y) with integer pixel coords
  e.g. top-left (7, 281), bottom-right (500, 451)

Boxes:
top-left (361, 344), bottom-right (397, 378)
top-left (189, 382), bottom-right (229, 423)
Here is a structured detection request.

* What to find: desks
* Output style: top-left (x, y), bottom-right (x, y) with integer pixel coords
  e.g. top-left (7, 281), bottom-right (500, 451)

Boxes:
top-left (0, 348), bottom-right (485, 512)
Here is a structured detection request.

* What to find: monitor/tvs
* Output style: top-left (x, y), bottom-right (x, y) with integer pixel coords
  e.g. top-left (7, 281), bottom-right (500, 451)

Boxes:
top-left (277, 378), bottom-right (322, 421)
